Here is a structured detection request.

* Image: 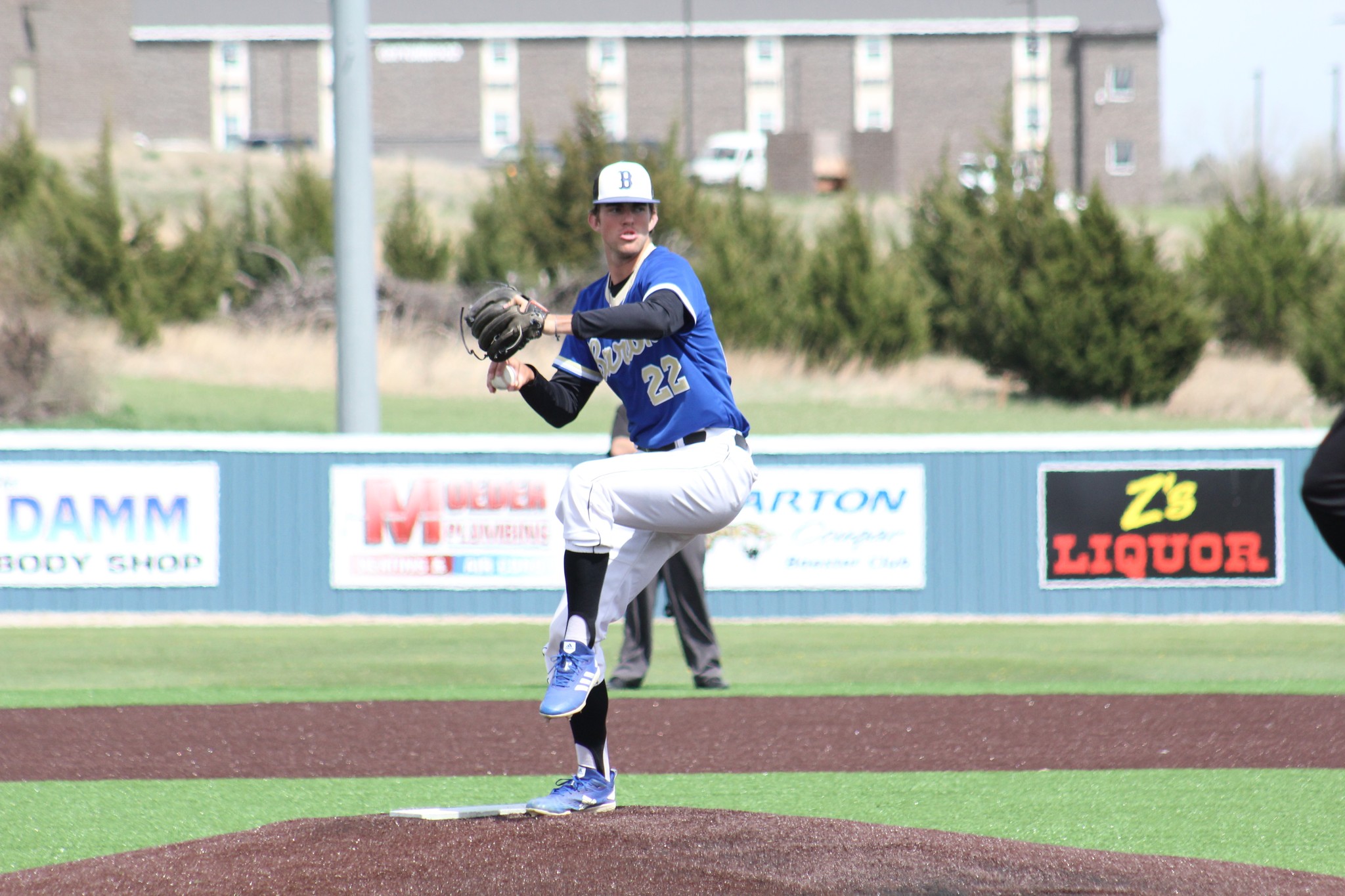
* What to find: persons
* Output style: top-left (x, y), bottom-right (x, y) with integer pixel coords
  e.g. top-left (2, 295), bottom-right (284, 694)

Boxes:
top-left (609, 395), bottom-right (734, 691)
top-left (484, 158), bottom-right (755, 816)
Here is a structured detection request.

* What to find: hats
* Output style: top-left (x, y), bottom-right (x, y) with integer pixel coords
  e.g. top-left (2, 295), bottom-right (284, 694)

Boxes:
top-left (593, 161), bottom-right (660, 203)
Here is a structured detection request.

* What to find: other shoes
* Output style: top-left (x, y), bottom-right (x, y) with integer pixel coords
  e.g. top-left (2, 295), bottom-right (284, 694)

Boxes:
top-left (698, 677), bottom-right (728, 688)
top-left (609, 677), bottom-right (642, 689)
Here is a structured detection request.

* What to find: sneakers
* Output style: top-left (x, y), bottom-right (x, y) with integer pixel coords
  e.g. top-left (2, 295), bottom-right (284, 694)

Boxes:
top-left (525, 765), bottom-right (617, 815)
top-left (539, 640), bottom-right (600, 722)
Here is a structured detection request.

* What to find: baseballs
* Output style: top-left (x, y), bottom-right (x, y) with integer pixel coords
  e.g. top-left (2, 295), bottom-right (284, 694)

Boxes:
top-left (491, 365), bottom-right (517, 391)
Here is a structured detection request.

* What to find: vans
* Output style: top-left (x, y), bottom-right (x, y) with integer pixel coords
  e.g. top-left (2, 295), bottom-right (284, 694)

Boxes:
top-left (683, 130), bottom-right (769, 192)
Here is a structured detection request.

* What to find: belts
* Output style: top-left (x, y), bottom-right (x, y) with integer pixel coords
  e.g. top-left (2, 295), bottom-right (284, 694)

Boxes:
top-left (637, 431), bottom-right (750, 452)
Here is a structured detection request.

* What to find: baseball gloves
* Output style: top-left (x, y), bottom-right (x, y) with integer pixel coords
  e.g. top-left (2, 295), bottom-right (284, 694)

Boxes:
top-left (458, 281), bottom-right (552, 363)
